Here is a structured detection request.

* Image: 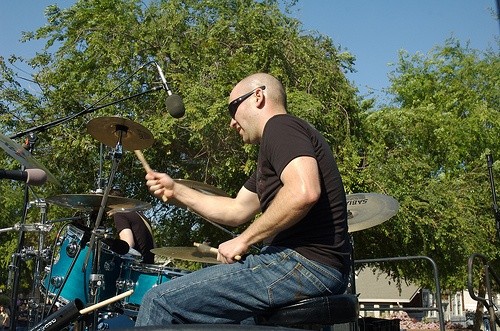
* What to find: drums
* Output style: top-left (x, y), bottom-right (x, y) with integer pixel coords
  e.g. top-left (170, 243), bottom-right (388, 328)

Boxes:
top-left (81, 307), bottom-right (138, 331)
top-left (41, 223), bottom-right (143, 312)
top-left (113, 264), bottom-right (190, 307)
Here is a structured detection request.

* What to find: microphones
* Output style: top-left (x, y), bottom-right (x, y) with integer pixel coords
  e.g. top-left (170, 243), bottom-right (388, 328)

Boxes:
top-left (97, 237), bottom-right (130, 255)
top-left (155, 62), bottom-right (185, 118)
top-left (83, 210), bottom-right (107, 221)
top-left (0, 168), bottom-right (47, 185)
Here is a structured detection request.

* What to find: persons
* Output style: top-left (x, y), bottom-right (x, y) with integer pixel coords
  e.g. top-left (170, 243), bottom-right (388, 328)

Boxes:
top-left (133, 73), bottom-right (350, 328)
top-left (109, 189), bottom-right (154, 264)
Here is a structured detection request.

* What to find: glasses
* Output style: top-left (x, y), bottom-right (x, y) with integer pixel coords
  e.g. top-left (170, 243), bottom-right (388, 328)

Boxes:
top-left (229, 86), bottom-right (266, 120)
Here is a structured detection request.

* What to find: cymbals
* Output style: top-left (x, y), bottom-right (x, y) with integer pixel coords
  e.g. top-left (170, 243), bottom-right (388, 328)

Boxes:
top-left (47, 194), bottom-right (151, 213)
top-left (148, 179), bottom-right (232, 210)
top-left (86, 117), bottom-right (154, 150)
top-left (150, 246), bottom-right (222, 264)
top-left (0, 134), bottom-right (53, 178)
top-left (345, 193), bottom-right (400, 234)
